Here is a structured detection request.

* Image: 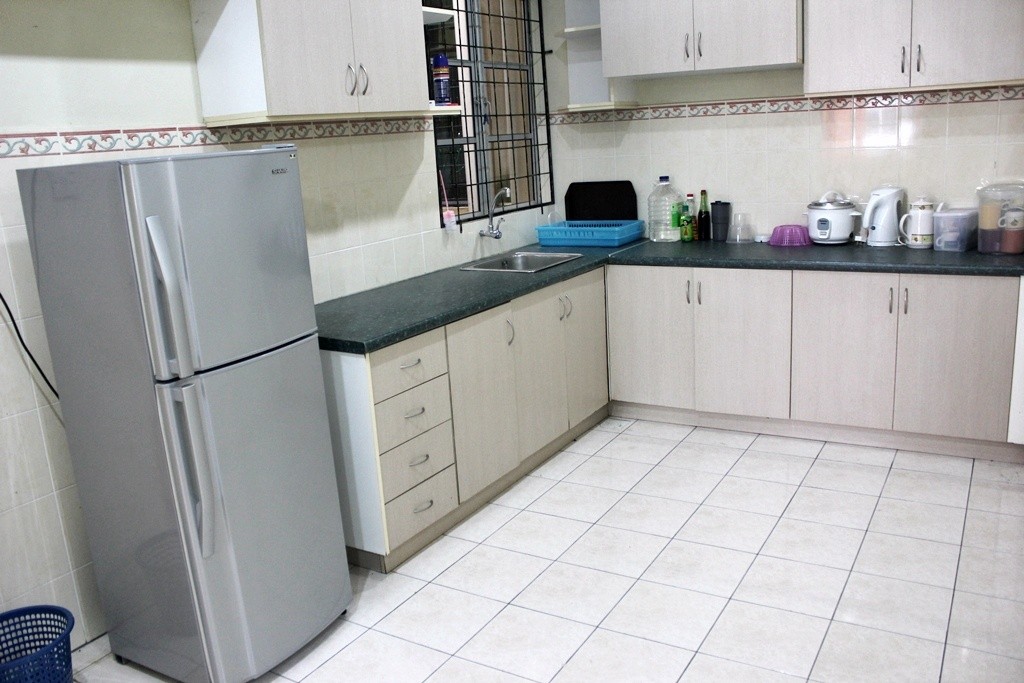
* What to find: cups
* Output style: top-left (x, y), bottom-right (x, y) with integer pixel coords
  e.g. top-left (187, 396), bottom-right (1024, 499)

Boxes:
top-left (711, 201), bottom-right (730, 241)
top-left (978, 197), bottom-right (1023, 253)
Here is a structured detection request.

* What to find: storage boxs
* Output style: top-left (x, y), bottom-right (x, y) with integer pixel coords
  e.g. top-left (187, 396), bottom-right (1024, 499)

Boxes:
top-left (931, 205), bottom-right (982, 255)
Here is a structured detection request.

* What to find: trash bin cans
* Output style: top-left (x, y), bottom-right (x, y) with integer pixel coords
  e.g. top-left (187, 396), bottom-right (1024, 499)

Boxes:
top-left (0, 605), bottom-right (74, 683)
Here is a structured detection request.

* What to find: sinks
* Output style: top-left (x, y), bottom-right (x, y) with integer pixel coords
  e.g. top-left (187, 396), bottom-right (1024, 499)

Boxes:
top-left (458, 252), bottom-right (585, 274)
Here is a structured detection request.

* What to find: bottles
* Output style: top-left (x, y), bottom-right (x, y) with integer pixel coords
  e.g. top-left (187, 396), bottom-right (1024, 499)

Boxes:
top-left (432, 52), bottom-right (452, 105)
top-left (698, 189), bottom-right (710, 241)
top-left (685, 194), bottom-right (698, 241)
top-left (679, 205), bottom-right (693, 243)
top-left (647, 175), bottom-right (684, 242)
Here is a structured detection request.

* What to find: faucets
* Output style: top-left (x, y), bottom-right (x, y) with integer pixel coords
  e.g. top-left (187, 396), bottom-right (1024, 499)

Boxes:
top-left (480, 186), bottom-right (512, 240)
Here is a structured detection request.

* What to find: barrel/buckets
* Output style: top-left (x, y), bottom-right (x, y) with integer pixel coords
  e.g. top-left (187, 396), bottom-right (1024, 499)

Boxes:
top-left (976, 180), bottom-right (1024, 254)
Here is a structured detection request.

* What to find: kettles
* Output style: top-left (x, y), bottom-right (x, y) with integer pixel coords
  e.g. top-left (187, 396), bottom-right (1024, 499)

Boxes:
top-left (863, 183), bottom-right (905, 246)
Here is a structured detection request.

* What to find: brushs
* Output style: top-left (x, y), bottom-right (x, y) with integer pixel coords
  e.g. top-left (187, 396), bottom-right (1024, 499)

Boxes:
top-left (440, 170), bottom-right (457, 234)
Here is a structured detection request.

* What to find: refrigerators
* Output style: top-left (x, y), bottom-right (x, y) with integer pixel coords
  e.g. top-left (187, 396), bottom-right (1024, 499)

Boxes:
top-left (16, 143), bottom-right (355, 683)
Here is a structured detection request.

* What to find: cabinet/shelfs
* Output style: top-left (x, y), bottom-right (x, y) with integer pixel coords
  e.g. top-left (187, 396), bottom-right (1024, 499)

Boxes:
top-left (602, 262), bottom-right (793, 439)
top-left (802, 0), bottom-right (1024, 100)
top-left (513, 262), bottom-right (611, 481)
top-left (563, 22), bottom-right (640, 113)
top-left (789, 268), bottom-right (1022, 462)
top-left (444, 298), bottom-right (525, 522)
top-left (188, 0), bottom-right (432, 129)
top-left (318, 323), bottom-right (461, 577)
top-left (422, 6), bottom-right (463, 118)
top-left (598, 0), bottom-right (804, 79)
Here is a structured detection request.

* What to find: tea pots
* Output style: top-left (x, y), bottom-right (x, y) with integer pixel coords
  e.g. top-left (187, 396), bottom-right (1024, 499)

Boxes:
top-left (899, 196), bottom-right (944, 249)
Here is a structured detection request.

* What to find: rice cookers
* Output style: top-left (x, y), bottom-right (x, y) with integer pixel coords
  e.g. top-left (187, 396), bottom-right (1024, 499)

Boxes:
top-left (801, 190), bottom-right (862, 245)
